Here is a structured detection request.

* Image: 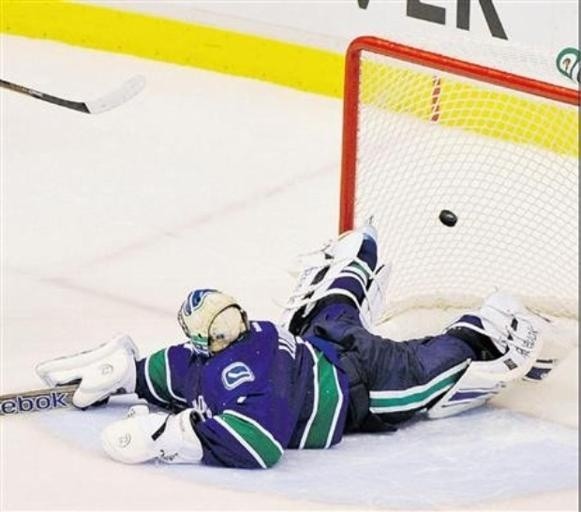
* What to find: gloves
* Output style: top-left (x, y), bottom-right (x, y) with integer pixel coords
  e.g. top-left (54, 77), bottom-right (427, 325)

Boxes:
top-left (35, 332), bottom-right (140, 408)
top-left (99, 404), bottom-right (206, 464)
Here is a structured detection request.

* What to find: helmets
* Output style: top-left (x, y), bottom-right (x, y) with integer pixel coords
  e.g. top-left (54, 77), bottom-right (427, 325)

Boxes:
top-left (177, 289), bottom-right (251, 358)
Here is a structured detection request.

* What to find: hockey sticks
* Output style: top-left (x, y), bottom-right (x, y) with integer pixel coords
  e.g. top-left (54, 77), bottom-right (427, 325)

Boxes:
top-left (0, 74), bottom-right (146, 115)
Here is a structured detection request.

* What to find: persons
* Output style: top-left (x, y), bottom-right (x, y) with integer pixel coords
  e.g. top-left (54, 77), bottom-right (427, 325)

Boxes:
top-left (33, 214), bottom-right (573, 470)
